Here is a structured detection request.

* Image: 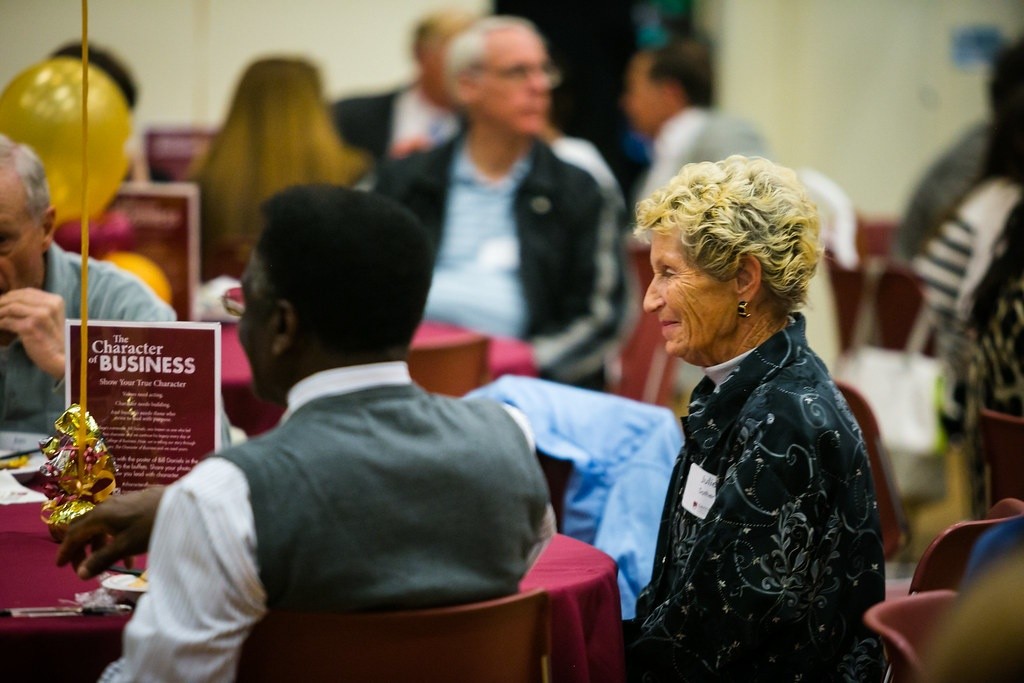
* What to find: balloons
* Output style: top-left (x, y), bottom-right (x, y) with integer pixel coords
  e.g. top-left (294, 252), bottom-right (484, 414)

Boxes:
top-left (0, 58), bottom-right (132, 233)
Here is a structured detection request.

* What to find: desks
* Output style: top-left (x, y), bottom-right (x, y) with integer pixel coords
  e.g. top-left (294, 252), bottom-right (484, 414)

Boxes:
top-left (0, 428), bottom-right (621, 683)
top-left (199, 283), bottom-right (534, 447)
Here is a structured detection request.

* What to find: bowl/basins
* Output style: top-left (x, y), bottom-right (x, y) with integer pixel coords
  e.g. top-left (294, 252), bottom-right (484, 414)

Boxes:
top-left (101, 572), bottom-right (146, 606)
top-left (0, 430), bottom-right (52, 484)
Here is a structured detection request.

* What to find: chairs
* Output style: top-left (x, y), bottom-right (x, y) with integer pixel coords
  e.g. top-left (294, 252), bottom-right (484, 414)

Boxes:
top-left (837, 380), bottom-right (1024, 683)
top-left (236, 589), bottom-right (544, 683)
top-left (402, 338), bottom-right (492, 401)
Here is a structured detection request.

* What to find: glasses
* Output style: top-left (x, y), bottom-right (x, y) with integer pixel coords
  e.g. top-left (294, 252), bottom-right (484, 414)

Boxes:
top-left (221, 286), bottom-right (250, 318)
top-left (469, 62), bottom-right (557, 80)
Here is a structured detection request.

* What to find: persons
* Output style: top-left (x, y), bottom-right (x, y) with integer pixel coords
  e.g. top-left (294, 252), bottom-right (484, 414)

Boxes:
top-left (45, 41), bottom-right (146, 184)
top-left (622, 152), bottom-right (885, 683)
top-left (904, 37), bottom-right (1024, 466)
top-left (191, 56), bottom-right (365, 287)
top-left (0, 135), bottom-right (177, 437)
top-left (622, 38), bottom-right (756, 239)
top-left (56, 185), bottom-right (555, 683)
top-left (329, 18), bottom-right (631, 379)
